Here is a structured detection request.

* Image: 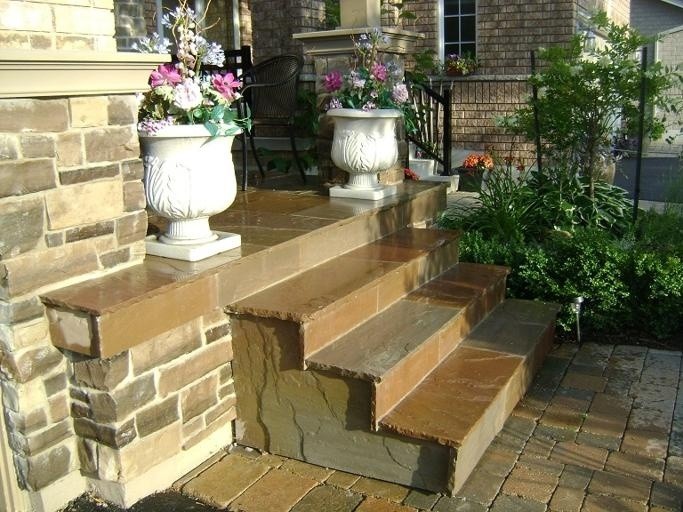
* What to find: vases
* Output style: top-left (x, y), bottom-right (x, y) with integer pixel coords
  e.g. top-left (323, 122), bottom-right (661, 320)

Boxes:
top-left (446, 68), bottom-right (465, 77)
top-left (326, 110), bottom-right (404, 200)
top-left (135, 123), bottom-right (243, 263)
top-left (456, 167), bottom-right (488, 193)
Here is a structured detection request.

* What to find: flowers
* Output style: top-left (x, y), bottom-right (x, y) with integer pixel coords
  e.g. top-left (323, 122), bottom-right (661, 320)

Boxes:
top-left (461, 153), bottom-right (493, 169)
top-left (444, 54), bottom-right (466, 72)
top-left (318, 27), bottom-right (411, 109)
top-left (128, 1), bottom-right (253, 138)
